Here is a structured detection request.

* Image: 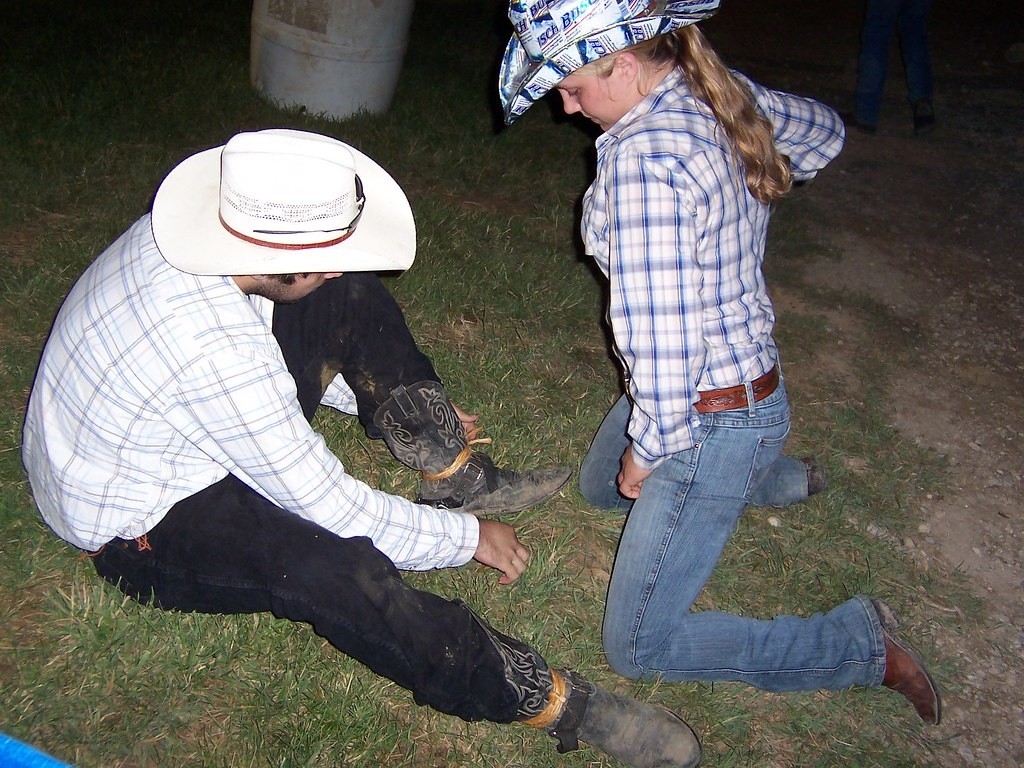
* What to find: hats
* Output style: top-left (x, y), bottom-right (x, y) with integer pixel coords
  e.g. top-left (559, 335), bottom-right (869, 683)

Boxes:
top-left (499, 0), bottom-right (720, 126)
top-left (152, 129), bottom-right (416, 275)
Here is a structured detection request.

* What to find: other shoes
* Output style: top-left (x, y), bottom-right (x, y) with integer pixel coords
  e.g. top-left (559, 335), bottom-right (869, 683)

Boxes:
top-left (839, 112), bottom-right (875, 134)
top-left (914, 97), bottom-right (935, 137)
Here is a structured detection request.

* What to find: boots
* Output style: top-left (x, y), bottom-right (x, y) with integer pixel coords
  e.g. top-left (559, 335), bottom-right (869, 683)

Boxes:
top-left (451, 598), bottom-right (702, 768)
top-left (373, 380), bottom-right (573, 518)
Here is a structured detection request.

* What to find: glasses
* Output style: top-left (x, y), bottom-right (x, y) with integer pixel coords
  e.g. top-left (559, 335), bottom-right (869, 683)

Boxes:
top-left (253, 174), bottom-right (366, 234)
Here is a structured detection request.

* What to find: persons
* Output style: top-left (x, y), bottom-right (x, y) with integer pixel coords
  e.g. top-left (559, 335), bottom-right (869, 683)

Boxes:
top-left (22, 129), bottom-right (701, 768)
top-left (498, 0), bottom-right (942, 726)
top-left (842, 0), bottom-right (936, 136)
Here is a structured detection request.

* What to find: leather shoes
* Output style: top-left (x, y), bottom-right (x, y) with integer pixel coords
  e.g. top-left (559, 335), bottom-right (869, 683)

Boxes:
top-left (800, 454), bottom-right (825, 495)
top-left (865, 595), bottom-right (941, 725)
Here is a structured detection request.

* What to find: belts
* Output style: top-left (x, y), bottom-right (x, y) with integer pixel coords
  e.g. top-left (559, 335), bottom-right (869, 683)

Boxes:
top-left (622, 358), bottom-right (779, 413)
